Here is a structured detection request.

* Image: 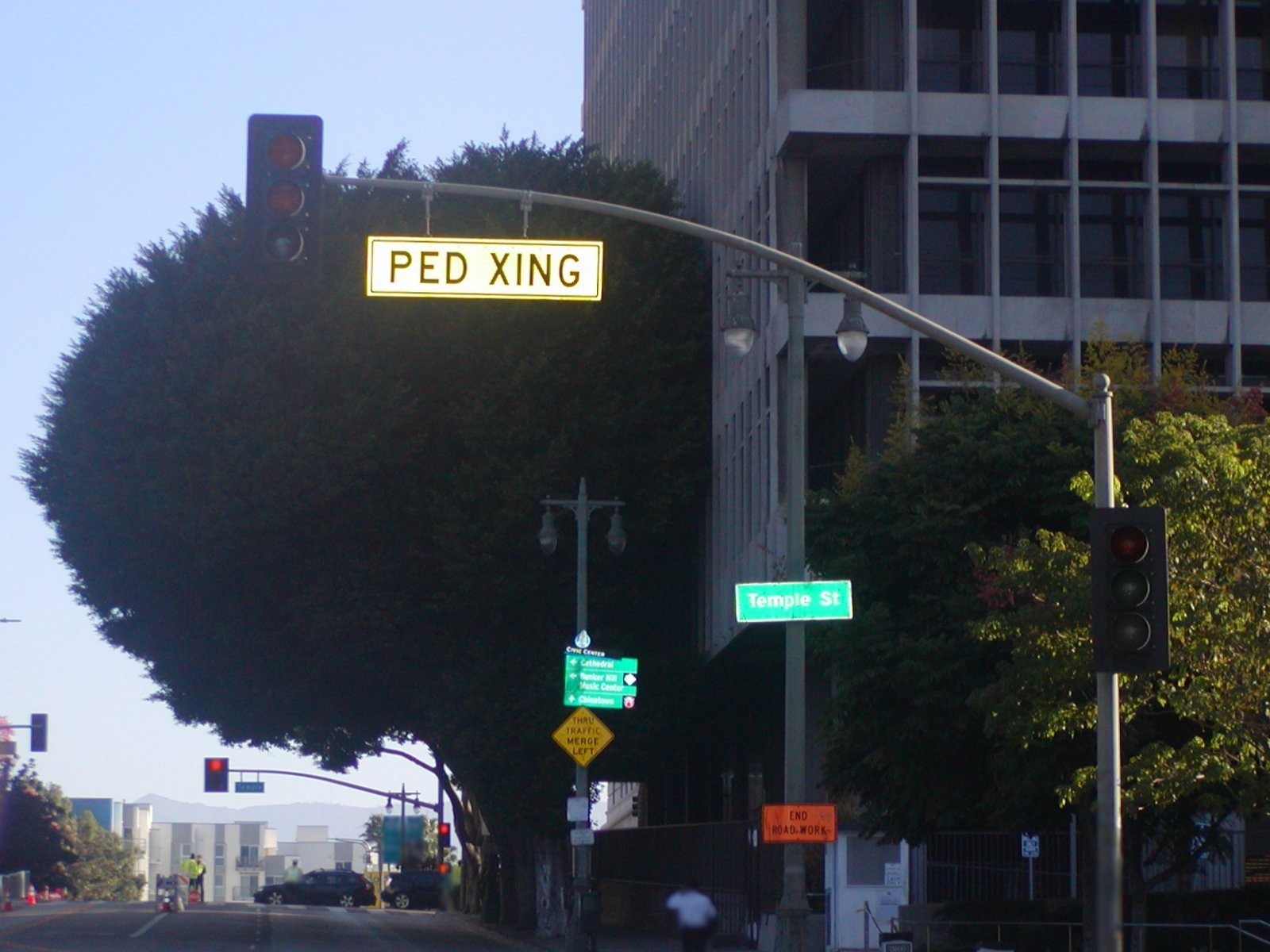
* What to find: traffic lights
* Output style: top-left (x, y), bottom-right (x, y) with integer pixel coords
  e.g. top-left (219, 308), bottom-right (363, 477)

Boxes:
top-left (438, 823), bottom-right (450, 846)
top-left (204, 757), bottom-right (228, 793)
top-left (1093, 505), bottom-right (1168, 671)
top-left (438, 863), bottom-right (453, 874)
top-left (246, 113), bottom-right (325, 283)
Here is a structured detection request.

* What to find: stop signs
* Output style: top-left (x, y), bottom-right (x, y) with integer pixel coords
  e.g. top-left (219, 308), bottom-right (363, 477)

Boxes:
top-left (189, 891), bottom-right (201, 903)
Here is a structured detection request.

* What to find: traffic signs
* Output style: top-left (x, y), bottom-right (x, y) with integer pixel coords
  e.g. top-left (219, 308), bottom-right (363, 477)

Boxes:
top-left (562, 654), bottom-right (639, 710)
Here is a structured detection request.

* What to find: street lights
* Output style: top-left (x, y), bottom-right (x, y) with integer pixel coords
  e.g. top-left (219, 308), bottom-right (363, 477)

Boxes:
top-left (327, 836), bottom-right (372, 873)
top-left (715, 241), bottom-right (873, 952)
top-left (385, 784), bottom-right (422, 873)
top-left (537, 475), bottom-right (628, 952)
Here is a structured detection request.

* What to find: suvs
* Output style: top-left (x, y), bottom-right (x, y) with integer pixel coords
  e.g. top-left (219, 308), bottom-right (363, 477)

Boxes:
top-left (381, 871), bottom-right (460, 910)
top-left (254, 868), bottom-right (377, 908)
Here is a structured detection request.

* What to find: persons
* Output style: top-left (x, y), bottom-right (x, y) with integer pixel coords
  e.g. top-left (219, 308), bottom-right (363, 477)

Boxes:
top-left (667, 875), bottom-right (717, 952)
top-left (282, 860), bottom-right (302, 883)
top-left (185, 853), bottom-right (206, 903)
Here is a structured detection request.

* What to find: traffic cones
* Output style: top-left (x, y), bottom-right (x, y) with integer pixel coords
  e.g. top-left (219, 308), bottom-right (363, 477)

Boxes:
top-left (23, 884), bottom-right (38, 906)
top-left (0, 890), bottom-right (14, 912)
top-left (159, 889), bottom-right (174, 913)
